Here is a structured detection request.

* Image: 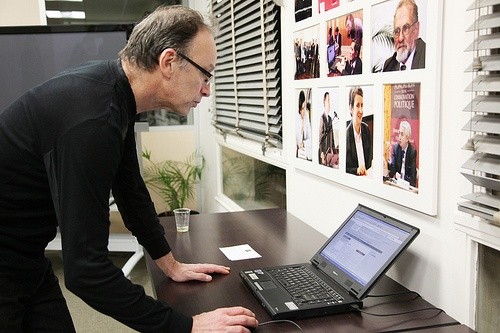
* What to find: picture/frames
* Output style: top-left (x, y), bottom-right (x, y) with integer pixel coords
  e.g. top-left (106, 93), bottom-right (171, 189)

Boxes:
top-left (283, 0), bottom-right (441, 216)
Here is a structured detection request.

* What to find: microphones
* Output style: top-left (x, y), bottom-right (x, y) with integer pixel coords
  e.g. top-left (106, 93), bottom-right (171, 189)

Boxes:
top-left (383, 52), bottom-right (397, 73)
top-left (334, 111), bottom-right (339, 120)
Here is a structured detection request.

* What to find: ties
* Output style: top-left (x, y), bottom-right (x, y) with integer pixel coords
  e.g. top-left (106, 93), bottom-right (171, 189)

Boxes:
top-left (401, 65), bottom-right (406, 70)
top-left (402, 151), bottom-right (405, 158)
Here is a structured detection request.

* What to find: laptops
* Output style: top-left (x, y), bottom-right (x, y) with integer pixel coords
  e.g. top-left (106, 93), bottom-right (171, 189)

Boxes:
top-left (238, 205), bottom-right (420, 322)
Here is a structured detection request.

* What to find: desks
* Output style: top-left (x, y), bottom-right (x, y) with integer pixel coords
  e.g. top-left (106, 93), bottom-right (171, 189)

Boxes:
top-left (144, 209), bottom-right (477, 333)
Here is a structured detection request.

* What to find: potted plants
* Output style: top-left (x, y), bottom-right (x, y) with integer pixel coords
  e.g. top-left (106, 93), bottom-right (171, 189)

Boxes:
top-left (143, 153), bottom-right (203, 217)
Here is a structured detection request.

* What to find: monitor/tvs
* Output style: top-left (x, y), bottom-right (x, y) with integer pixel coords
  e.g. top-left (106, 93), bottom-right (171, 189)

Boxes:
top-left (327, 42), bottom-right (335, 66)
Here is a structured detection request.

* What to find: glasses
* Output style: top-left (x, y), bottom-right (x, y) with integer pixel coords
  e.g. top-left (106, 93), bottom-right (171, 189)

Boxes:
top-left (176, 52), bottom-right (214, 86)
top-left (393, 22), bottom-right (416, 38)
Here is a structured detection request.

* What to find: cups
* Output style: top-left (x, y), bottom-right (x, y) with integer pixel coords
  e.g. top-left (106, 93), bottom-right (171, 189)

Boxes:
top-left (173, 207), bottom-right (192, 232)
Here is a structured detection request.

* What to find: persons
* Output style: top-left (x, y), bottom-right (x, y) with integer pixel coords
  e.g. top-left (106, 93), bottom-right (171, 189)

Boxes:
top-left (383, 121), bottom-right (416, 187)
top-left (345, 87), bottom-right (372, 176)
top-left (0, 4), bottom-right (258, 333)
top-left (295, 43), bottom-right (320, 80)
top-left (319, 92), bottom-right (339, 165)
top-left (296, 90), bottom-right (310, 158)
top-left (382, 0), bottom-right (426, 72)
top-left (328, 13), bottom-right (363, 76)
